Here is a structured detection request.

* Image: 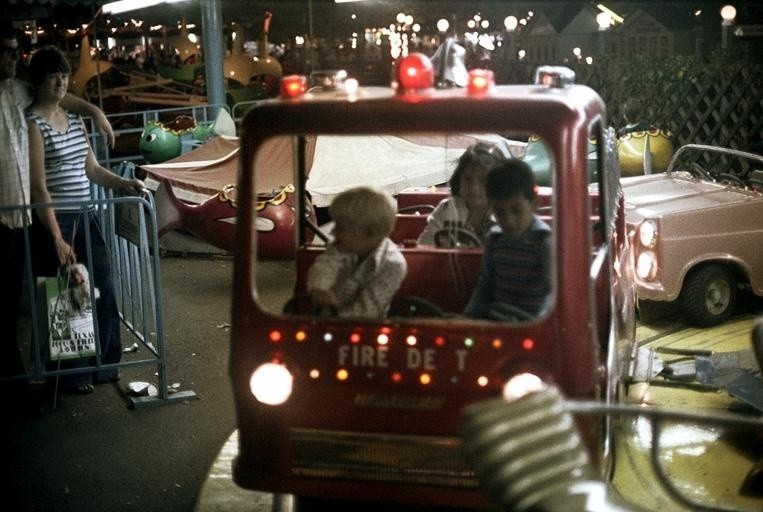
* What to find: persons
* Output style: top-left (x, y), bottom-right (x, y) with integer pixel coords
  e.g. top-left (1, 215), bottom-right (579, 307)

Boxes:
top-left (442, 155), bottom-right (554, 326)
top-left (304, 185), bottom-right (410, 320)
top-left (21, 44), bottom-right (150, 395)
top-left (415, 142), bottom-right (508, 247)
top-left (2, 24), bottom-right (37, 390)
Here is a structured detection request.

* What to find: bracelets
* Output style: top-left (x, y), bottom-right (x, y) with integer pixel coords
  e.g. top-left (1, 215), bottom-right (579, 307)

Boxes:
top-left (115, 176), bottom-right (127, 193)
